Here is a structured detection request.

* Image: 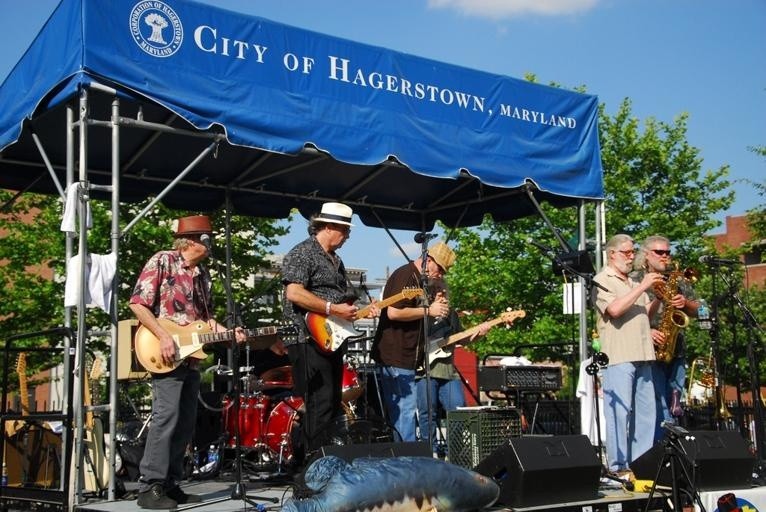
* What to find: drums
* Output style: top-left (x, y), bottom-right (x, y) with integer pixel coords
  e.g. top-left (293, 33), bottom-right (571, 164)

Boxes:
top-left (246, 364), bottom-right (296, 392)
top-left (220, 394), bottom-right (277, 452)
top-left (340, 359), bottom-right (365, 405)
top-left (265, 395), bottom-right (357, 466)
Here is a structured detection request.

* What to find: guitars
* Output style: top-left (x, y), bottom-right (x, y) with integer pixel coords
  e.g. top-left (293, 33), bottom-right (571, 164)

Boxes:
top-left (417, 304), bottom-right (526, 374)
top-left (85, 356), bottom-right (109, 493)
top-left (305, 286), bottom-right (425, 354)
top-left (135, 316), bottom-right (300, 375)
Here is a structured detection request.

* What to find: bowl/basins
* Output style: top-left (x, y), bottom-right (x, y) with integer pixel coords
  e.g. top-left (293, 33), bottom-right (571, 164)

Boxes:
top-left (633, 479), bottom-right (653, 493)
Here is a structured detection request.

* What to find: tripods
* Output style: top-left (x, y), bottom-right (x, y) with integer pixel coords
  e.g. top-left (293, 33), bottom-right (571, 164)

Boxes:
top-left (168, 377), bottom-right (279, 512)
top-left (645, 456), bottom-right (707, 512)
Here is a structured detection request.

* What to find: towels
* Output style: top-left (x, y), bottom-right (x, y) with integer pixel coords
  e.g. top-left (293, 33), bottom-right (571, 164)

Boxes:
top-left (60, 182), bottom-right (93, 237)
top-left (63, 252), bottom-right (118, 315)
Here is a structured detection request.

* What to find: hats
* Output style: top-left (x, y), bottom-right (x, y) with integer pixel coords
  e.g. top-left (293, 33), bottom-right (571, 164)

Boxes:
top-left (313, 201), bottom-right (357, 228)
top-left (428, 240), bottom-right (455, 274)
top-left (171, 214), bottom-right (218, 236)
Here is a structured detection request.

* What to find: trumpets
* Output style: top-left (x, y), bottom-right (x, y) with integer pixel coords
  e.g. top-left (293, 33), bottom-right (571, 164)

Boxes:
top-left (699, 341), bottom-right (734, 420)
top-left (632, 265), bottom-right (700, 284)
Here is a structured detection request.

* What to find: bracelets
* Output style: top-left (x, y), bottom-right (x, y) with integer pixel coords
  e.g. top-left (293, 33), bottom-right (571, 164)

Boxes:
top-left (326, 301), bottom-right (331, 315)
top-left (427, 307), bottom-right (430, 316)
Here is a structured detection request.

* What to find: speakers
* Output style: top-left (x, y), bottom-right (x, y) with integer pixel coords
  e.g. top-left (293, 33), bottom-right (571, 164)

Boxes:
top-left (629, 431), bottom-right (752, 490)
top-left (473, 435), bottom-right (603, 509)
top-left (294, 440), bottom-right (431, 501)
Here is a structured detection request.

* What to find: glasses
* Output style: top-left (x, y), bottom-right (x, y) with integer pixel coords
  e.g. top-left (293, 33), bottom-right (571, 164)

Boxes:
top-left (649, 249), bottom-right (670, 256)
top-left (620, 249), bottom-right (637, 255)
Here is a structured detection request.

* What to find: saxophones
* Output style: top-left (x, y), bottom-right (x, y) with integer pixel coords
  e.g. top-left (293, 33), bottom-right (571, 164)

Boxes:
top-left (656, 261), bottom-right (689, 362)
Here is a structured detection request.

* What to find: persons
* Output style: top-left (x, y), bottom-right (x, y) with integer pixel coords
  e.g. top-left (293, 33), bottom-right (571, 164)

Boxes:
top-left (129, 216), bottom-right (246, 509)
top-left (417, 278), bottom-right (491, 458)
top-left (370, 241), bottom-right (456, 444)
top-left (590, 234), bottom-right (667, 478)
top-left (627, 232), bottom-right (706, 445)
top-left (283, 203), bottom-right (381, 467)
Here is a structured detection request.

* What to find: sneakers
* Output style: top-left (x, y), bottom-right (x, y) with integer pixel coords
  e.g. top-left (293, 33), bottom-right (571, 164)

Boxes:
top-left (137, 486), bottom-right (179, 510)
top-left (167, 486), bottom-right (202, 504)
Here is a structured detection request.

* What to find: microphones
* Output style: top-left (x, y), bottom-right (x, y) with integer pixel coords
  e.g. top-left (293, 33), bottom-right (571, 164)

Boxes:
top-left (699, 255), bottom-right (738, 266)
top-left (527, 237), bottom-right (547, 251)
top-left (356, 278), bottom-right (364, 301)
top-left (201, 234), bottom-right (213, 256)
top-left (414, 233), bottom-right (439, 243)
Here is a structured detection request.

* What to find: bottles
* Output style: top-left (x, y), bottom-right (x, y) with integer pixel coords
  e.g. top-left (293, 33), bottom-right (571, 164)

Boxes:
top-left (698, 298), bottom-right (712, 329)
top-left (192, 445), bottom-right (219, 467)
